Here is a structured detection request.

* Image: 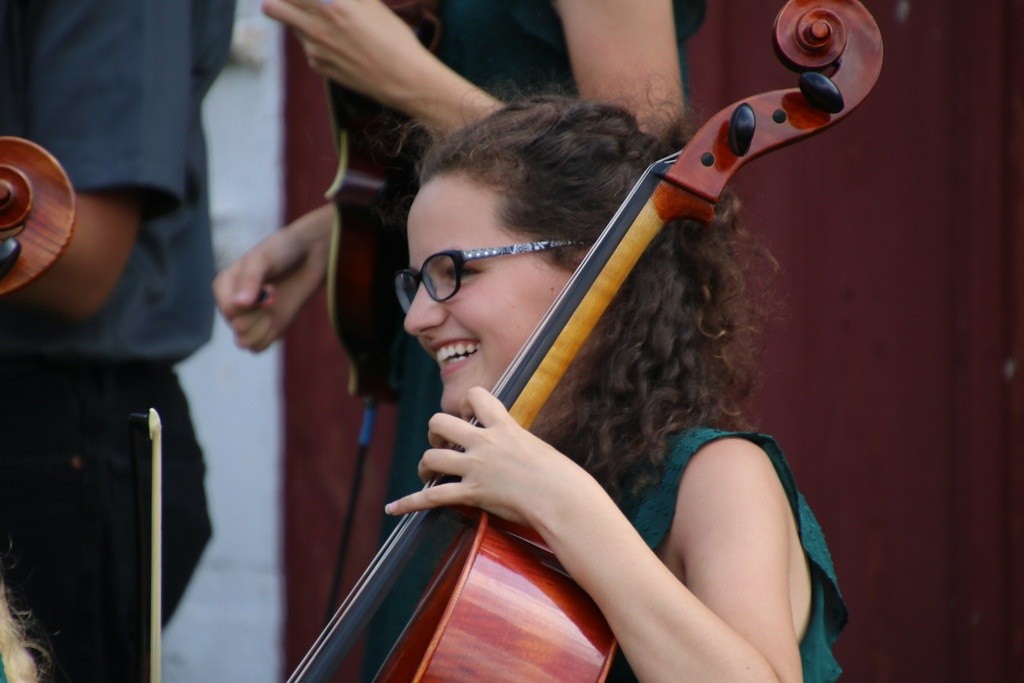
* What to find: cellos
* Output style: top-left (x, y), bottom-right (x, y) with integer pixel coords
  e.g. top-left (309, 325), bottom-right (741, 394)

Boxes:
top-left (280, 0), bottom-right (893, 683)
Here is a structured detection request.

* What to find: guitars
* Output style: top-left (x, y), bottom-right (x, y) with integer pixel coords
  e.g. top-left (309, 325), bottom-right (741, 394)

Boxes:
top-left (314, 1), bottom-right (434, 402)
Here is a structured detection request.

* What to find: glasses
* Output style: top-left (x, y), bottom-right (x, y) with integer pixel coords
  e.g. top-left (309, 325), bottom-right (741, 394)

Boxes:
top-left (395, 238), bottom-right (596, 315)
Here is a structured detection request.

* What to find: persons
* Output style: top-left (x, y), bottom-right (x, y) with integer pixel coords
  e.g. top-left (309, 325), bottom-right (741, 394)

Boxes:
top-left (385, 94), bottom-right (850, 683)
top-left (0, 0), bottom-right (238, 683)
top-left (213, 0), bottom-right (707, 553)
